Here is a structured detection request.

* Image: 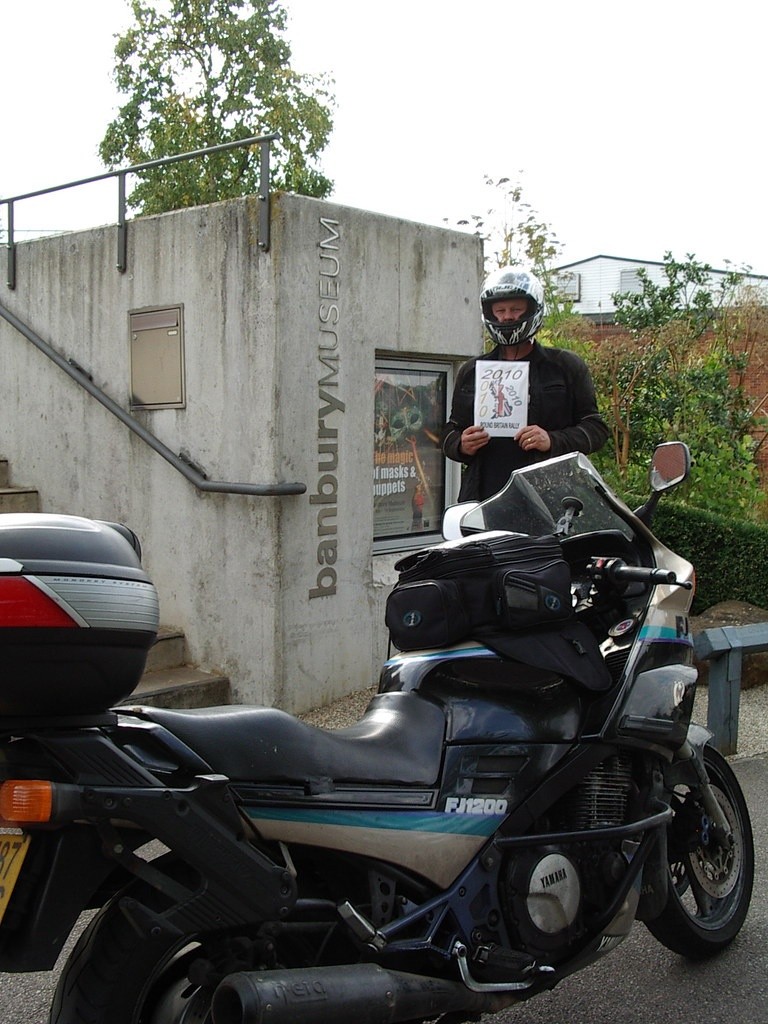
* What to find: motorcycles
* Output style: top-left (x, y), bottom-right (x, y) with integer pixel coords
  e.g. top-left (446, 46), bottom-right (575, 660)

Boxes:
top-left (1, 442), bottom-right (755, 1023)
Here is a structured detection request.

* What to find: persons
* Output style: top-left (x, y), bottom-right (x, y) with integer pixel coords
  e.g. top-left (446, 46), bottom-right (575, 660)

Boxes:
top-left (439, 267), bottom-right (615, 537)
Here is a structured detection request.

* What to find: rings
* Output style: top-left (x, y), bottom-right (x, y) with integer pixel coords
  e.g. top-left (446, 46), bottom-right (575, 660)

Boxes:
top-left (527, 438), bottom-right (531, 444)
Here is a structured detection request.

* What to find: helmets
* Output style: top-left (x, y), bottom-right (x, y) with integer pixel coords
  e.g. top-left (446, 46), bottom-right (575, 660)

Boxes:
top-left (479, 267), bottom-right (545, 345)
